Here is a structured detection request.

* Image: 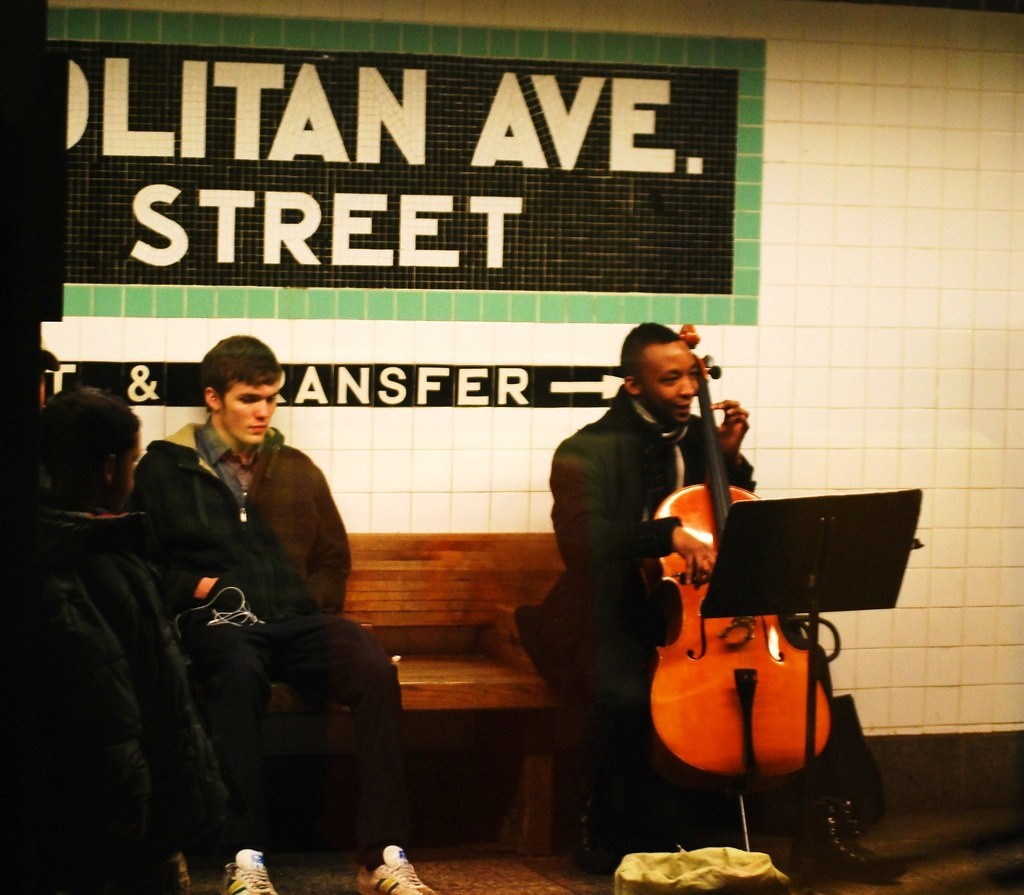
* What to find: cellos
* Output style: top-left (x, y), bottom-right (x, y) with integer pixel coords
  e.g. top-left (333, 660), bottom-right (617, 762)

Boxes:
top-left (647, 320), bottom-right (838, 853)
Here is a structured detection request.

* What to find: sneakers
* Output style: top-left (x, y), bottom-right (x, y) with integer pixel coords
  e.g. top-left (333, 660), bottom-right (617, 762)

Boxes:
top-left (355, 844), bottom-right (437, 895)
top-left (221, 849), bottom-right (279, 895)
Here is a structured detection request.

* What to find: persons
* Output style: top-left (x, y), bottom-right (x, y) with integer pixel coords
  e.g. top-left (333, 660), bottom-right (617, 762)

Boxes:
top-left (134, 338), bottom-right (436, 895)
top-left (0, 391), bottom-right (230, 895)
top-left (515, 323), bottom-right (906, 882)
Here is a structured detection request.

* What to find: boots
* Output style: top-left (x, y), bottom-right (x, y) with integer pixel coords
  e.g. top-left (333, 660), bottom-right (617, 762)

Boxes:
top-left (575, 792), bottom-right (617, 874)
top-left (787, 800), bottom-right (906, 883)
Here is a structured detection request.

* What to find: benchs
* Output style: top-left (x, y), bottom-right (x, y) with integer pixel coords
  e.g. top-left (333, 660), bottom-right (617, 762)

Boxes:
top-left (341, 532), bottom-right (568, 857)
